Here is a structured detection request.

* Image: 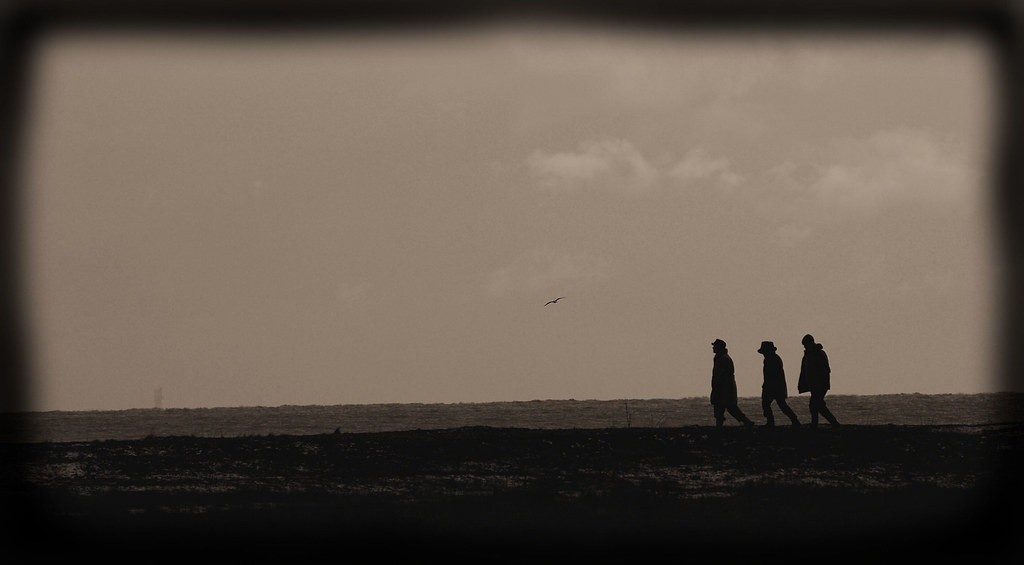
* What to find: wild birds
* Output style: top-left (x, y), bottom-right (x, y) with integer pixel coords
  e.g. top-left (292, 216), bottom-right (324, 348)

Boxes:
top-left (544, 296), bottom-right (567, 307)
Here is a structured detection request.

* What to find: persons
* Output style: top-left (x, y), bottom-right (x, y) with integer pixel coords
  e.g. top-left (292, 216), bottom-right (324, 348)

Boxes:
top-left (758, 341), bottom-right (801, 426)
top-left (798, 334), bottom-right (840, 424)
top-left (710, 339), bottom-right (755, 428)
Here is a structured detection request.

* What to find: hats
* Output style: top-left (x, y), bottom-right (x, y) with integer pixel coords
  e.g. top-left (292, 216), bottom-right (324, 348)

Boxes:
top-left (801, 334), bottom-right (814, 345)
top-left (712, 339), bottom-right (726, 347)
top-left (757, 341), bottom-right (777, 353)
